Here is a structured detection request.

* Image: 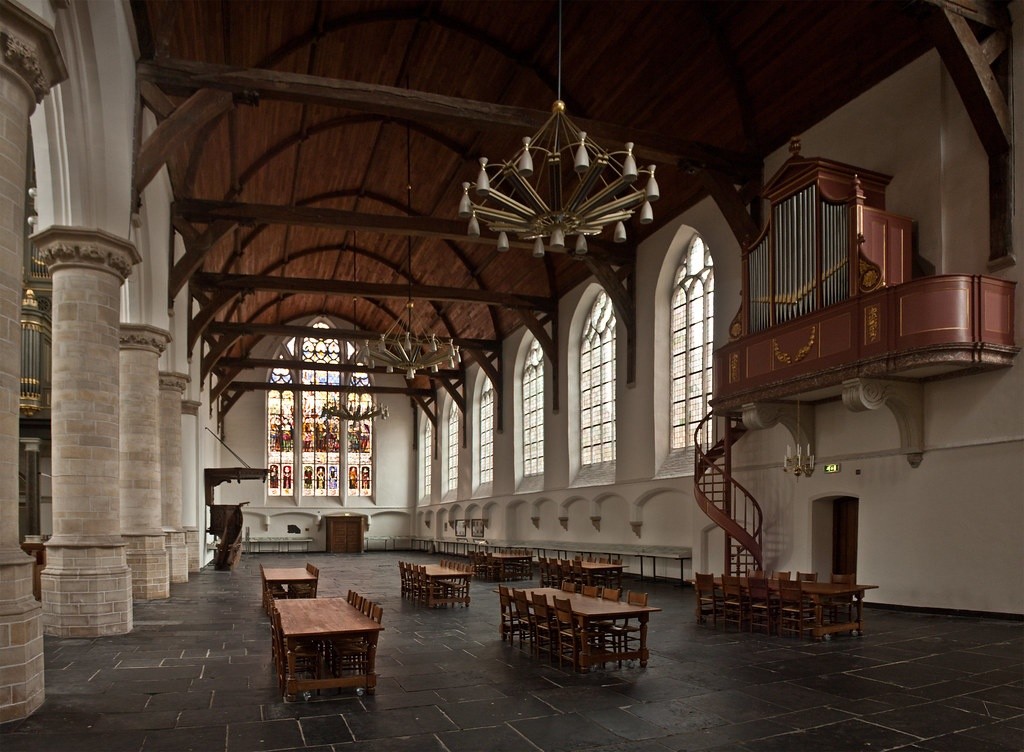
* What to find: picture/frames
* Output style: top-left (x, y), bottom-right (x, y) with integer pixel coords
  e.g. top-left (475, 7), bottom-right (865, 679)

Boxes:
top-left (471, 518), bottom-right (484, 537)
top-left (455, 519), bottom-right (466, 536)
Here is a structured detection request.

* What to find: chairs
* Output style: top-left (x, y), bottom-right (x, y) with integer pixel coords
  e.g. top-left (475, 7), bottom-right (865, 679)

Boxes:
top-left (498, 583), bottom-right (533, 646)
top-left (553, 595), bottom-right (605, 673)
top-left (778, 571), bottom-right (855, 639)
top-left (600, 589), bottom-right (648, 666)
top-left (326, 598), bottom-right (372, 674)
top-left (346, 589), bottom-right (356, 605)
top-left (329, 604), bottom-right (383, 689)
top-left (585, 586), bottom-right (622, 658)
top-left (512, 586), bottom-right (552, 654)
top-left (324, 593), bottom-right (363, 660)
top-left (537, 555), bottom-right (622, 587)
top-left (570, 584), bottom-right (600, 650)
top-left (547, 580), bottom-right (578, 626)
top-left (721, 574), bottom-right (754, 629)
top-left (257, 562), bottom-right (322, 700)
top-left (748, 577), bottom-right (783, 635)
top-left (531, 591), bottom-right (575, 662)
top-left (398, 548), bottom-right (475, 609)
top-left (694, 571), bottom-right (725, 627)
top-left (476, 549), bottom-right (535, 582)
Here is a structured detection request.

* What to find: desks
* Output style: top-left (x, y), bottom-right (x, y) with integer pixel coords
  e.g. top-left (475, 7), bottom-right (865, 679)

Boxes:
top-left (402, 564), bottom-right (473, 608)
top-left (687, 577), bottom-right (879, 641)
top-left (263, 568), bottom-right (317, 606)
top-left (482, 553), bottom-right (536, 582)
top-left (546, 560), bottom-right (630, 592)
top-left (508, 588), bottom-right (663, 671)
top-left (273, 597), bottom-right (385, 701)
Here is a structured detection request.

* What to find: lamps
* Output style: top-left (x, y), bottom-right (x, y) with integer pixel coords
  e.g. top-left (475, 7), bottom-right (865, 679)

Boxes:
top-left (457, 0), bottom-right (660, 259)
top-left (321, 266), bottom-right (390, 429)
top-left (354, 126), bottom-right (462, 379)
top-left (783, 397), bottom-right (816, 483)
